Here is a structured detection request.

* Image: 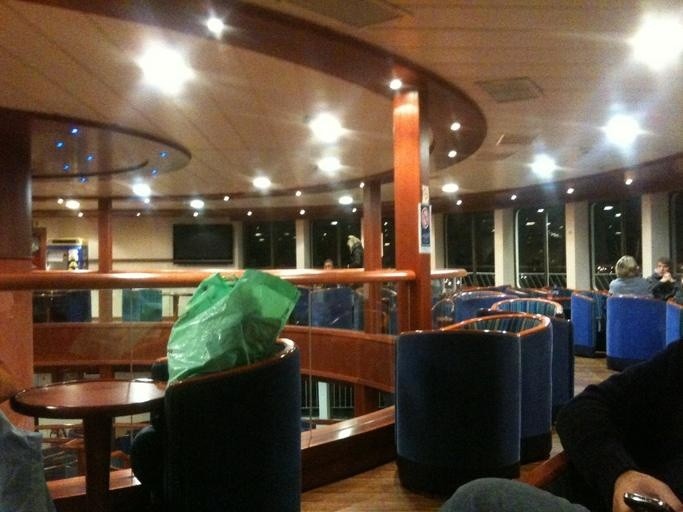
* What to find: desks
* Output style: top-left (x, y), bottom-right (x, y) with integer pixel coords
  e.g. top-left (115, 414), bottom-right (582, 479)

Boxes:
top-left (9, 377), bottom-right (167, 512)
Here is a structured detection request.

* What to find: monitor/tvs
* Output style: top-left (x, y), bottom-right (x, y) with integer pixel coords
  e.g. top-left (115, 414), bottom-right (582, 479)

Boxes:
top-left (173, 223), bottom-right (233, 264)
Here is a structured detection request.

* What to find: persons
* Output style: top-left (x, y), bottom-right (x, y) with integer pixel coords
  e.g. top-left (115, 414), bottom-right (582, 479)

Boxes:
top-left (312, 258), bottom-right (340, 289)
top-left (345, 234), bottom-right (363, 268)
top-left (435, 339), bottom-right (683, 512)
top-left (608, 255), bottom-right (683, 304)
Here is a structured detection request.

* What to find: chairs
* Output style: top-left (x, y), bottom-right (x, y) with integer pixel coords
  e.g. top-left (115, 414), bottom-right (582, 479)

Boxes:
top-left (128, 337), bottom-right (301, 511)
top-left (289, 283), bottom-right (395, 336)
top-left (395, 284), bottom-right (683, 499)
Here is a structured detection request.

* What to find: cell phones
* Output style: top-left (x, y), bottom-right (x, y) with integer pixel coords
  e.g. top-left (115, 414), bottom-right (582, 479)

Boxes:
top-left (624, 492), bottom-right (676, 512)
